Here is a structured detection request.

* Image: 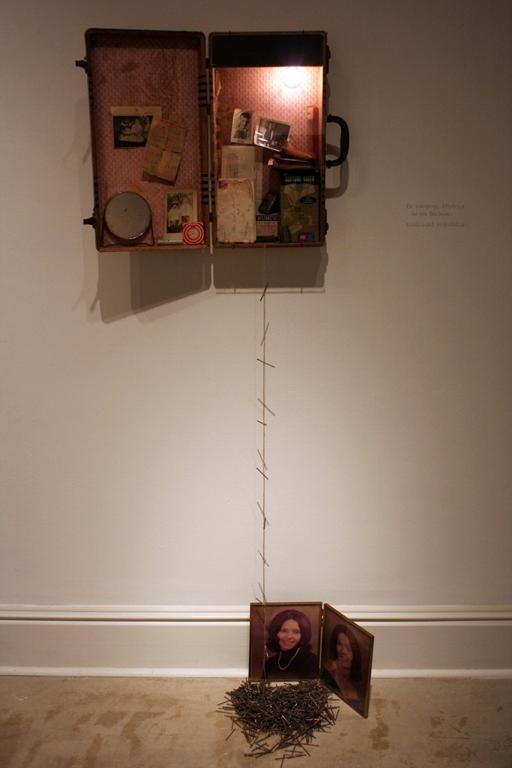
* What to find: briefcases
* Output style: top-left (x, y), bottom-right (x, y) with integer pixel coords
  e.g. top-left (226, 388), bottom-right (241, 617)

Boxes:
top-left (75, 27), bottom-right (351, 252)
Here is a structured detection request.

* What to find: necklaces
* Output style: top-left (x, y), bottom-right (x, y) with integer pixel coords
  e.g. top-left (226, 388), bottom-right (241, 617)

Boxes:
top-left (277, 648), bottom-right (301, 670)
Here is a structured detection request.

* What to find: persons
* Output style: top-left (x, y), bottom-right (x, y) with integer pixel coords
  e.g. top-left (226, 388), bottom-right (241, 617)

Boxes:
top-left (234, 112), bottom-right (250, 138)
top-left (262, 122), bottom-right (274, 145)
top-left (323, 623), bottom-right (364, 708)
top-left (264, 610), bottom-right (319, 678)
top-left (168, 196), bottom-right (191, 233)
top-left (118, 118), bottom-right (143, 136)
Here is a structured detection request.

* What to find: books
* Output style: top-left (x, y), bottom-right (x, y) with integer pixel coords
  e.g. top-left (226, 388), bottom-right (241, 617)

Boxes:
top-left (217, 144), bottom-right (264, 244)
